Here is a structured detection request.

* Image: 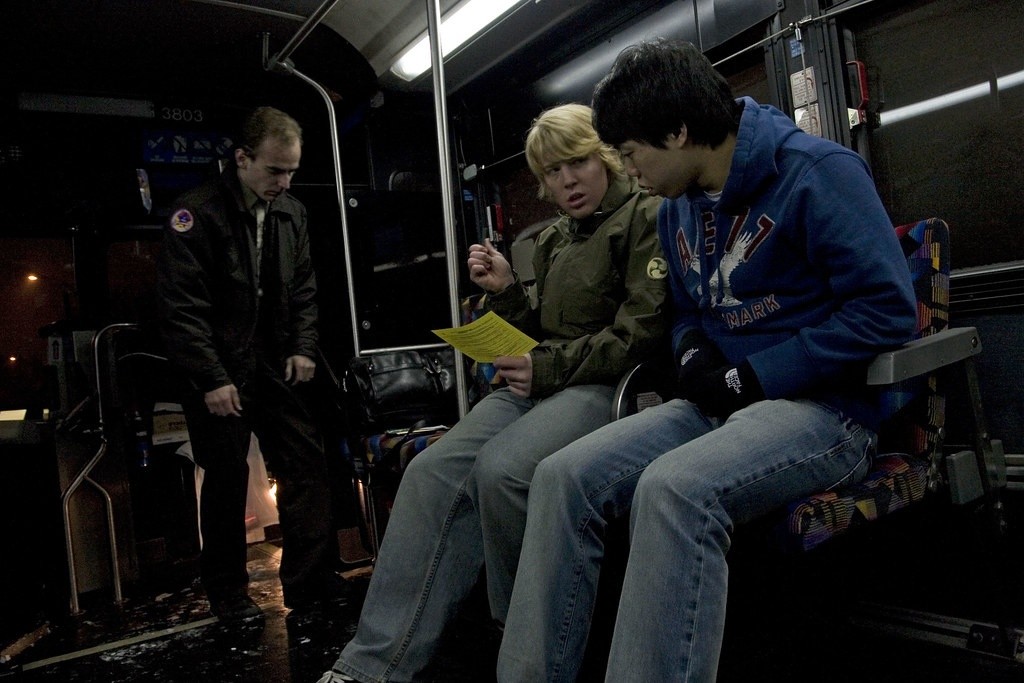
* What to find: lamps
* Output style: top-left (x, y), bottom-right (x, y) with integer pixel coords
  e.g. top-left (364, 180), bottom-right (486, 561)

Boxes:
top-left (389, 0), bottom-right (522, 81)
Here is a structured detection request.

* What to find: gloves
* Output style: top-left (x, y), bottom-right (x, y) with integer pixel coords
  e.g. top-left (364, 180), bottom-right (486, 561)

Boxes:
top-left (693, 357), bottom-right (765, 417)
top-left (675, 330), bottom-right (728, 383)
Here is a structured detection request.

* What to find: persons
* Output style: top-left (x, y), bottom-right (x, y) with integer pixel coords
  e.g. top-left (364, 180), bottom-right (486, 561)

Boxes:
top-left (316, 102), bottom-right (666, 683)
top-left (491, 41), bottom-right (919, 683)
top-left (160, 105), bottom-right (370, 631)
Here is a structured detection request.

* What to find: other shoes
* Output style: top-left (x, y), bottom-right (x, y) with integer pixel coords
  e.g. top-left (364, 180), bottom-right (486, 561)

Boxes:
top-left (282, 573), bottom-right (371, 612)
top-left (316, 671), bottom-right (355, 683)
top-left (208, 596), bottom-right (265, 631)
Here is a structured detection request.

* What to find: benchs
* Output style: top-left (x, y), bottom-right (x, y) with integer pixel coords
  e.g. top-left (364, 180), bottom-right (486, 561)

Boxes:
top-left (375, 216), bottom-right (1009, 552)
